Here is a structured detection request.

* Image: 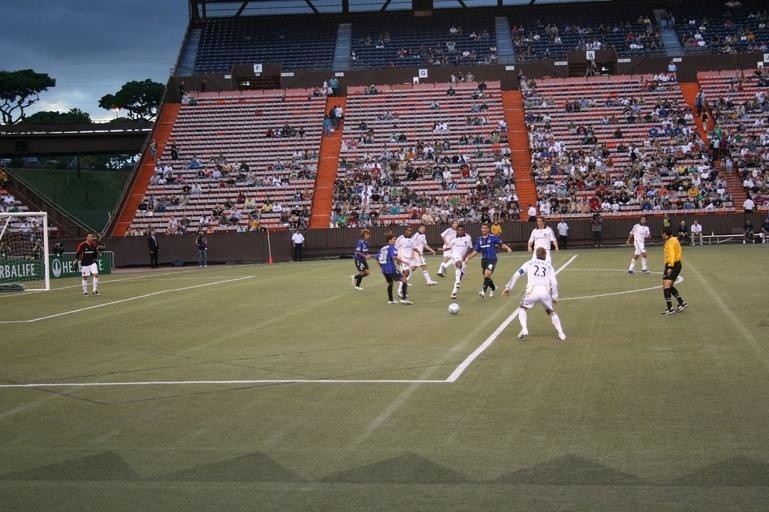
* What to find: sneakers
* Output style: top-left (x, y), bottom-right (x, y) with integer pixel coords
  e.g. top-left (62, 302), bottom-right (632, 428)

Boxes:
top-left (489, 287), bottom-right (498, 298)
top-left (676, 301), bottom-right (687, 312)
top-left (661, 307), bottom-right (675, 315)
top-left (354, 286), bottom-right (363, 291)
top-left (84, 290), bottom-right (99, 295)
top-left (479, 291), bottom-right (486, 298)
top-left (558, 334), bottom-right (567, 341)
top-left (518, 331), bottom-right (528, 339)
top-left (389, 299), bottom-right (398, 304)
top-left (643, 270), bottom-right (650, 274)
top-left (426, 272), bottom-right (460, 299)
top-left (351, 276), bottom-right (357, 286)
top-left (628, 270), bottom-right (634, 274)
top-left (397, 282), bottom-right (413, 304)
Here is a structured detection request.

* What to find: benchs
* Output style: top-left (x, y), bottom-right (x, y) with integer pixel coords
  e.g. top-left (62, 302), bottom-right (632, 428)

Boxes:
top-left (688, 234), bottom-right (769, 245)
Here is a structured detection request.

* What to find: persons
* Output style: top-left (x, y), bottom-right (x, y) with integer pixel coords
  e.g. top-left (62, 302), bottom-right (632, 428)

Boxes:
top-left (124, 9), bottom-right (769, 267)
top-left (75, 234), bottom-right (103, 296)
top-left (0, 184), bottom-right (64, 259)
top-left (350, 218), bottom-right (567, 341)
top-left (625, 214), bottom-right (687, 315)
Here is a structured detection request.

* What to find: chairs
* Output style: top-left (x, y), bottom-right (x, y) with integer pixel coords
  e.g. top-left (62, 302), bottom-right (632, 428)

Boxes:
top-left (0, 189), bottom-right (57, 241)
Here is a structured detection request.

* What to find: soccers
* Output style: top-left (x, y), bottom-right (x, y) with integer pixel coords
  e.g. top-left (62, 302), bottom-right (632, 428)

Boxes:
top-left (448, 303), bottom-right (459, 314)
